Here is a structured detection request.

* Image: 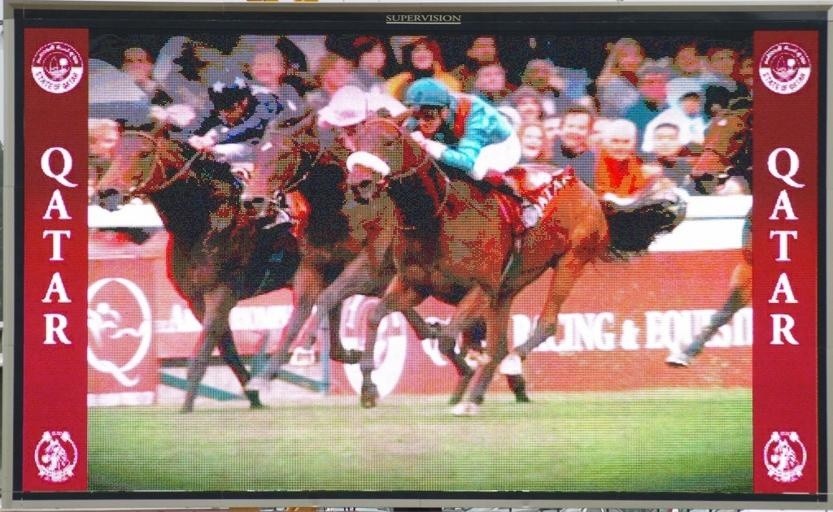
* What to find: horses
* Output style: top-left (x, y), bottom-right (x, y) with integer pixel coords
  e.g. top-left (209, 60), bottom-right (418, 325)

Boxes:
top-left (665, 100), bottom-right (754, 367)
top-left (346, 109), bottom-right (686, 417)
top-left (95, 119), bottom-right (364, 412)
top-left (239, 107), bottom-right (485, 392)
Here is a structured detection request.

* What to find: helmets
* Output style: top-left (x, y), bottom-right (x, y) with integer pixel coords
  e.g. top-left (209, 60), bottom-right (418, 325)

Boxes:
top-left (316, 85), bottom-right (368, 127)
top-left (403, 78), bottom-right (450, 107)
top-left (653, 190), bottom-right (684, 230)
top-left (207, 75), bottom-right (247, 108)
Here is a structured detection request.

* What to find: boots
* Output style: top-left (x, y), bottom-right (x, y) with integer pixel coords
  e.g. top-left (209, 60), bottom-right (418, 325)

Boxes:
top-left (496, 176), bottom-right (537, 230)
